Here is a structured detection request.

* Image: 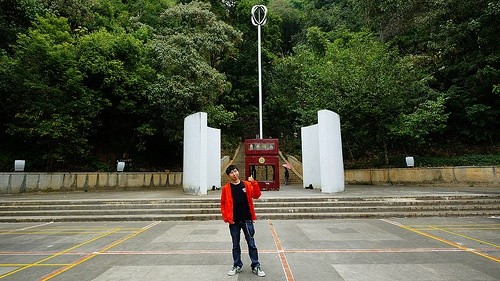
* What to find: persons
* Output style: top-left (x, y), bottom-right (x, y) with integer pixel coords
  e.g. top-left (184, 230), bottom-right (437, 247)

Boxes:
top-left (284, 168), bottom-right (289, 185)
top-left (220, 165), bottom-right (267, 277)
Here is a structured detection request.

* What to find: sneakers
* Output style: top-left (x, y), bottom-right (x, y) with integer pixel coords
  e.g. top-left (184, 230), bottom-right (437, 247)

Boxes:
top-left (228, 265), bottom-right (243, 276)
top-left (251, 266), bottom-right (265, 277)
